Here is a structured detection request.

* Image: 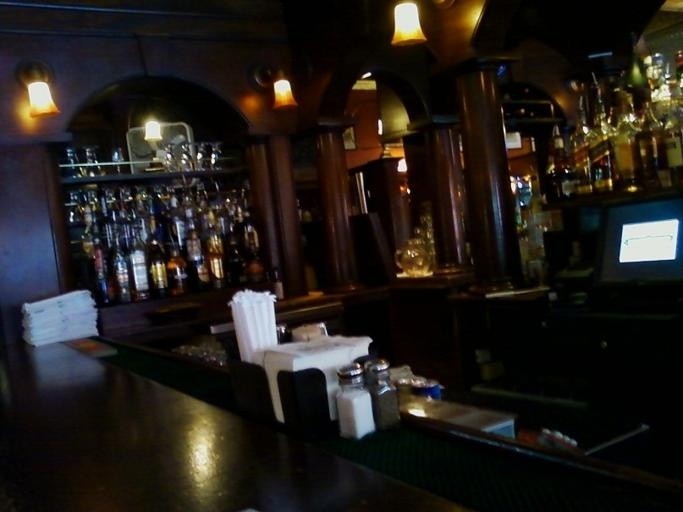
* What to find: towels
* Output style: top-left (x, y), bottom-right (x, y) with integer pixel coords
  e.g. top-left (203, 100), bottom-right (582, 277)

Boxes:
top-left (21, 290), bottom-right (100, 347)
top-left (226, 289), bottom-right (278, 367)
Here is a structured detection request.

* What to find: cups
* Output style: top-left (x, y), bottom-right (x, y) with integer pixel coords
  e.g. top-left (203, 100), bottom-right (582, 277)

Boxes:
top-left (66, 145), bottom-right (101, 179)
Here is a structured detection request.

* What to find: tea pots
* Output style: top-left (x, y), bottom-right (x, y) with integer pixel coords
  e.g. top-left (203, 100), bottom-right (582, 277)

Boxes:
top-left (395, 248), bottom-right (430, 277)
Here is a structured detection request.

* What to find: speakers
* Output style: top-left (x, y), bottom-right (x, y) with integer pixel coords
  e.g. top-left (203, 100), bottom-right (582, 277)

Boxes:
top-left (348, 212), bottom-right (397, 284)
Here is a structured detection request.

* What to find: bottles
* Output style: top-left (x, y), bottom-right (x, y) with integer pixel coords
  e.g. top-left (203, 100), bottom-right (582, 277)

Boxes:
top-left (550, 49), bottom-right (683, 198)
top-left (79, 184), bottom-right (284, 303)
top-left (335, 357), bottom-right (401, 443)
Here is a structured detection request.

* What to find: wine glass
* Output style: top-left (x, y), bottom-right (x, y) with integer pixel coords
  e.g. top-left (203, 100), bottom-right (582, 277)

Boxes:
top-left (161, 141), bottom-right (223, 172)
top-left (111, 147), bottom-right (125, 172)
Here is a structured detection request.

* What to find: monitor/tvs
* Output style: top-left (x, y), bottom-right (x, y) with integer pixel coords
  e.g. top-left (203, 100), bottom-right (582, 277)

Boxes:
top-left (587, 189), bottom-right (683, 288)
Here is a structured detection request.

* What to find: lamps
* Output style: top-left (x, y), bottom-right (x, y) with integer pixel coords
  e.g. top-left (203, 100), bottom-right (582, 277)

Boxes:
top-left (250, 59), bottom-right (298, 109)
top-left (18, 58), bottom-right (62, 117)
top-left (390, 0), bottom-right (455, 46)
top-left (144, 103), bottom-right (163, 142)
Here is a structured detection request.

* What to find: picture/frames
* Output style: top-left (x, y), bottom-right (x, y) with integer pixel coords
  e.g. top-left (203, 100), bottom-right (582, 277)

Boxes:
top-left (342, 126), bottom-right (356, 150)
